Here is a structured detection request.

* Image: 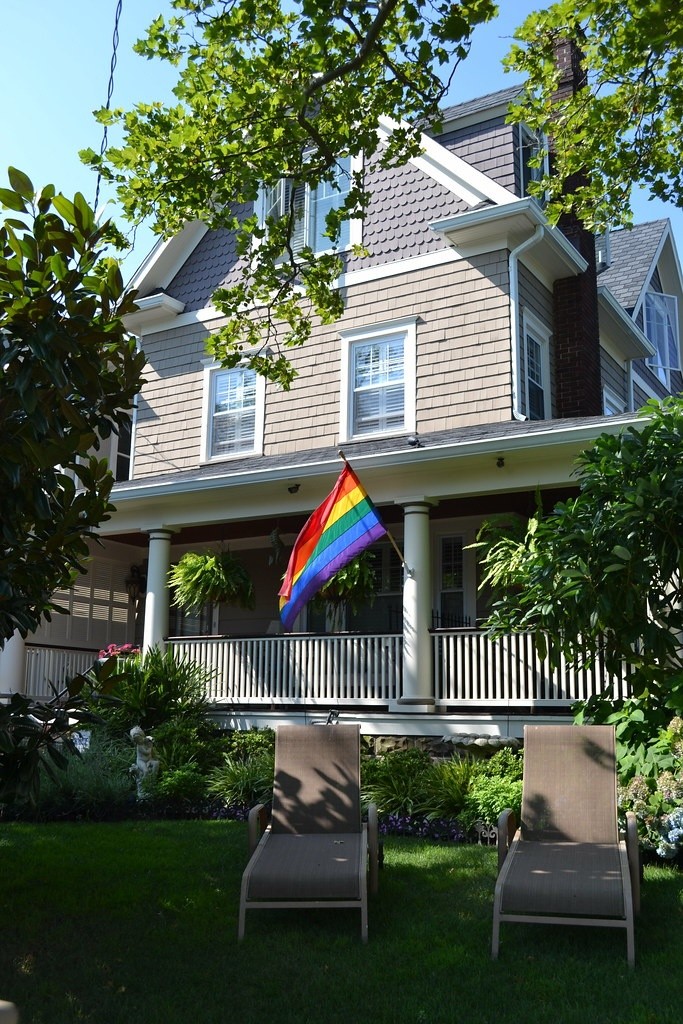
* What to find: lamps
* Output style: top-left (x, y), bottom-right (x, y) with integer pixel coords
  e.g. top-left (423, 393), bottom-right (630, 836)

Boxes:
top-left (288, 484), bottom-right (300, 494)
top-left (126, 564), bottom-right (147, 605)
top-left (496, 456), bottom-right (505, 468)
top-left (407, 436), bottom-right (425, 448)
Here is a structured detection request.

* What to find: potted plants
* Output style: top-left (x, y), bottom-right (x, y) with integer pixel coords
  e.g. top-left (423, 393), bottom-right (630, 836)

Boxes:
top-left (279, 550), bottom-right (378, 631)
top-left (163, 539), bottom-right (256, 619)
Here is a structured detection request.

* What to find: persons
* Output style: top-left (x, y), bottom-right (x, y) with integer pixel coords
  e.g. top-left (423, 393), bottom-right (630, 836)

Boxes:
top-left (129, 725), bottom-right (161, 802)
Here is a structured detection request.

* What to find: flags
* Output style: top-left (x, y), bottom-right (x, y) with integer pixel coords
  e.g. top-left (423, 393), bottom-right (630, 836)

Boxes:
top-left (276, 461), bottom-right (389, 635)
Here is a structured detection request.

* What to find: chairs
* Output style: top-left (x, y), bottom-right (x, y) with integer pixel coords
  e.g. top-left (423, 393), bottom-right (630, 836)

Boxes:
top-left (238, 724), bottom-right (377, 944)
top-left (491, 723), bottom-right (640, 970)
